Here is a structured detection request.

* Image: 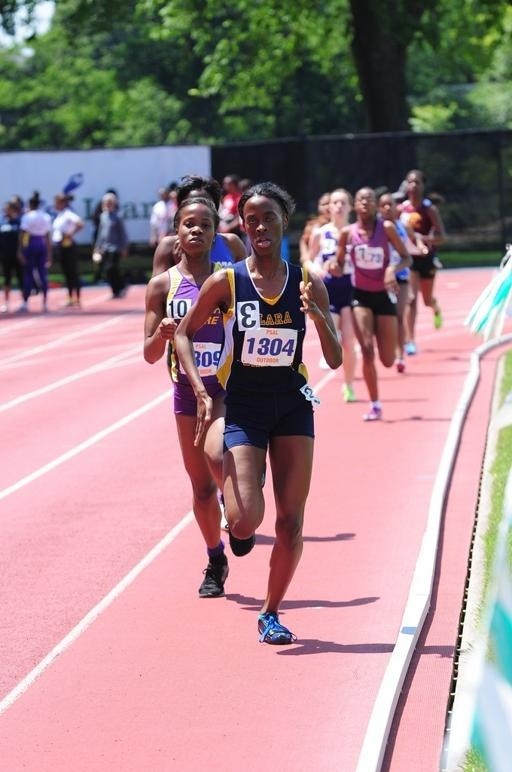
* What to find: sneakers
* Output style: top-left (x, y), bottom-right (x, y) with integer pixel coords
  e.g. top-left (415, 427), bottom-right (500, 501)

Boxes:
top-left (343, 384), bottom-right (355, 401)
top-left (363, 407), bottom-right (381, 421)
top-left (229, 533), bottom-right (255, 556)
top-left (199, 555), bottom-right (228, 597)
top-left (258, 611), bottom-right (291, 644)
top-left (434, 311), bottom-right (442, 328)
top-left (398, 343), bottom-right (415, 372)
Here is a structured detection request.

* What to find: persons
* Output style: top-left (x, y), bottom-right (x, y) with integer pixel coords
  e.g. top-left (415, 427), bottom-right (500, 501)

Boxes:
top-left (301, 169), bottom-right (451, 422)
top-left (143, 198), bottom-right (257, 599)
top-left (0, 175), bottom-right (256, 313)
top-left (171, 182), bottom-right (345, 644)
